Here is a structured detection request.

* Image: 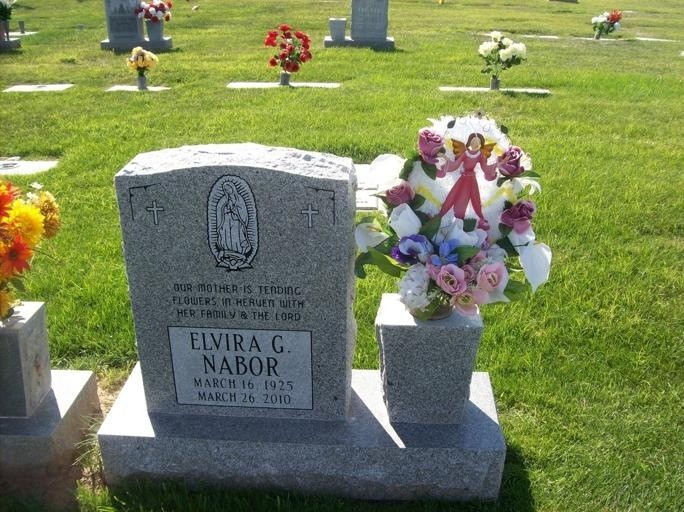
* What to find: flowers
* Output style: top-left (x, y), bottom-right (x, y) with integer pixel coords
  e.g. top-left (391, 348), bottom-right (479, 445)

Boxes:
top-left (125, 45), bottom-right (159, 77)
top-left (477, 30), bottom-right (528, 77)
top-left (134, 0), bottom-right (173, 23)
top-left (0, 0), bottom-right (18, 20)
top-left (354, 114), bottom-right (552, 317)
top-left (591, 8), bottom-right (623, 34)
top-left (265, 23), bottom-right (313, 71)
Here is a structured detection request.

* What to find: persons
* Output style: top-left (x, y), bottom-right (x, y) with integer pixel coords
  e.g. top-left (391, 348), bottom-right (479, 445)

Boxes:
top-left (216, 182), bottom-right (252, 269)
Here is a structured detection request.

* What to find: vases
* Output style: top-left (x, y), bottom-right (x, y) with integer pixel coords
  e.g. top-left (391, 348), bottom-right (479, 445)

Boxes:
top-left (280, 71), bottom-right (290, 84)
top-left (138, 77), bottom-right (146, 90)
top-left (0, 19), bottom-right (7, 41)
top-left (594, 31), bottom-right (601, 39)
top-left (144, 19), bottom-right (164, 40)
top-left (491, 76), bottom-right (499, 88)
top-left (409, 287), bottom-right (452, 320)
